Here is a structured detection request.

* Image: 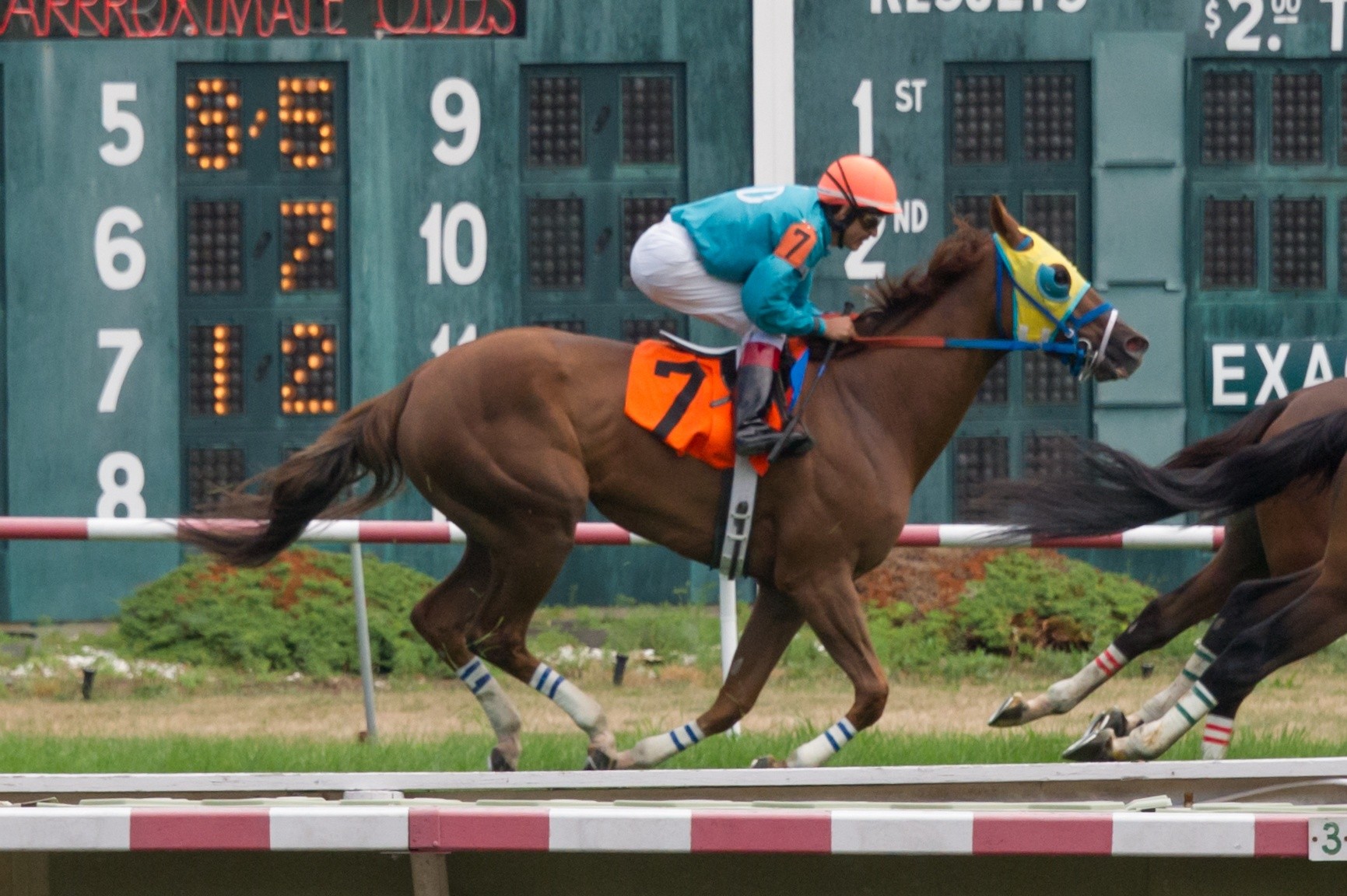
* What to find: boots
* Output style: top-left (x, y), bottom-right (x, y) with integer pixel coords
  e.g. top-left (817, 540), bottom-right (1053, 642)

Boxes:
top-left (732, 343), bottom-right (812, 457)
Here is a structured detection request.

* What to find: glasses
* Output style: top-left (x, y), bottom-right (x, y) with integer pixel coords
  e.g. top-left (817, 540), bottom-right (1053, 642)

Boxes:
top-left (864, 217), bottom-right (879, 230)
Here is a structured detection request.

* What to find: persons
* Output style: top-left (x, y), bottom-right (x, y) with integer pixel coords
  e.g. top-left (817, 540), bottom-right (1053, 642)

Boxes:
top-left (630, 154), bottom-right (901, 454)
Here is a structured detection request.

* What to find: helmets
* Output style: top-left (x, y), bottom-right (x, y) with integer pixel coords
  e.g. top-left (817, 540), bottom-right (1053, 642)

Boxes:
top-left (818, 153), bottom-right (900, 215)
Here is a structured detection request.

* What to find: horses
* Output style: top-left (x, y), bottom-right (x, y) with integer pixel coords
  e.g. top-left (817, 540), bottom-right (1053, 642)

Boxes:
top-left (988, 375), bottom-right (1346, 771)
top-left (158, 193), bottom-right (1151, 770)
